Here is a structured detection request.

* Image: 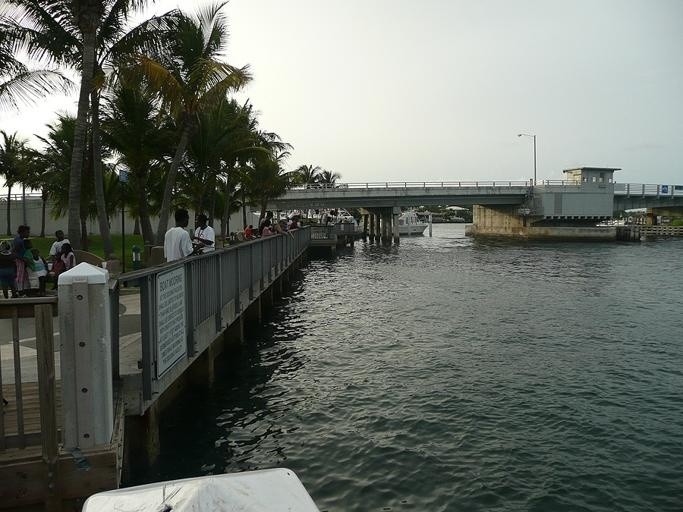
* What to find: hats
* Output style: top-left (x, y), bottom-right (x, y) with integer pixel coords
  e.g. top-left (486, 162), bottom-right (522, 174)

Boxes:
top-left (193, 214), bottom-right (211, 222)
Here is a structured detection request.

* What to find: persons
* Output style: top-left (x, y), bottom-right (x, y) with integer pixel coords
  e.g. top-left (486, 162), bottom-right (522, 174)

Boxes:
top-left (326, 217), bottom-right (334, 226)
top-left (243, 212), bottom-right (303, 240)
top-left (164, 208), bottom-right (205, 262)
top-left (191, 214), bottom-right (215, 255)
top-left (0, 225), bottom-right (76, 298)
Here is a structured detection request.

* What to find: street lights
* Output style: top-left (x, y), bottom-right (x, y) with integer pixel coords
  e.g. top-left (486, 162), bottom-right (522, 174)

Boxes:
top-left (517, 133), bottom-right (537, 186)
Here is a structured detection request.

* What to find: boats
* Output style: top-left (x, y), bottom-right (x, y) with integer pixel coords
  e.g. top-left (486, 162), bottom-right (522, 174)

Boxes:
top-left (366, 209), bottom-right (428, 236)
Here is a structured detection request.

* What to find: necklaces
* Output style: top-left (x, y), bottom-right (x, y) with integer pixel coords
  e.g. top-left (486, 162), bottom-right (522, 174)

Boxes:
top-left (198, 230), bottom-right (204, 242)
